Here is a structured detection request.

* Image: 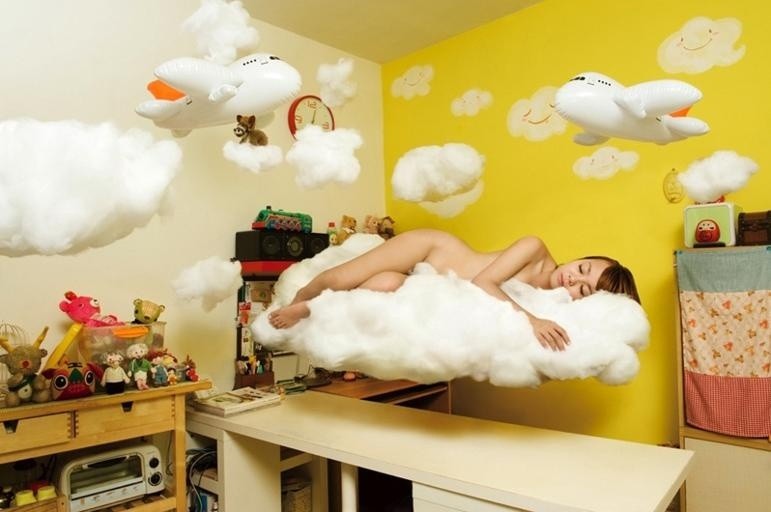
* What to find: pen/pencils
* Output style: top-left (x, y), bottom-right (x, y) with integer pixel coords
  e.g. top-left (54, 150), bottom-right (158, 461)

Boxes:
top-left (236, 351), bottom-right (273, 375)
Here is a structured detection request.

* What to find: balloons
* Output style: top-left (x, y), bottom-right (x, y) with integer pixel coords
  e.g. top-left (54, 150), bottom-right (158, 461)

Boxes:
top-left (554, 71), bottom-right (712, 145)
top-left (134, 52), bottom-right (302, 139)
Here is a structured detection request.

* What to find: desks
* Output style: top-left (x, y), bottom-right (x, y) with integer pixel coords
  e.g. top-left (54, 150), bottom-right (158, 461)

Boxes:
top-left (181, 370), bottom-right (698, 512)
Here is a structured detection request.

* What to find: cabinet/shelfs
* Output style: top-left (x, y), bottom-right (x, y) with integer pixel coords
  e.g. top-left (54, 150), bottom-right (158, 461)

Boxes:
top-left (1, 372), bottom-right (212, 512)
top-left (673, 243), bottom-right (771, 512)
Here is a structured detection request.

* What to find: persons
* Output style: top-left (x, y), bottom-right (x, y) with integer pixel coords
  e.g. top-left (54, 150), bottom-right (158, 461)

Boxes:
top-left (268, 228), bottom-right (641, 354)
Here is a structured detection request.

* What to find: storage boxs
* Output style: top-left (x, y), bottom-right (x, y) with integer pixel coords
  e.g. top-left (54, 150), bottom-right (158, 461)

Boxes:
top-left (74, 319), bottom-right (165, 366)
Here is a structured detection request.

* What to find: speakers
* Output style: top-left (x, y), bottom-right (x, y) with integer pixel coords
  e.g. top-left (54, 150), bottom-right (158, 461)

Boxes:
top-left (236, 230), bottom-right (331, 261)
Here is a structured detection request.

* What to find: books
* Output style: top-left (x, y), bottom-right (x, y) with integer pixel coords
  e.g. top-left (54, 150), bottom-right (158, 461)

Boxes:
top-left (195, 386), bottom-right (281, 417)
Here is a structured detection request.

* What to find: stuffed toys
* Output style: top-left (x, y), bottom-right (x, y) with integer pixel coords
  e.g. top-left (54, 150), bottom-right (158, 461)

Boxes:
top-left (327, 216), bottom-right (394, 244)
top-left (234, 115), bottom-right (268, 146)
top-left (0, 290), bottom-right (198, 407)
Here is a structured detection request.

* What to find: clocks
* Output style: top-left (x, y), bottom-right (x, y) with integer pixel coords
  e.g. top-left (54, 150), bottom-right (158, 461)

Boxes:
top-left (289, 93), bottom-right (334, 144)
